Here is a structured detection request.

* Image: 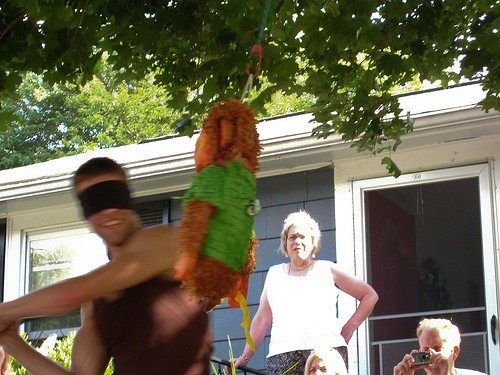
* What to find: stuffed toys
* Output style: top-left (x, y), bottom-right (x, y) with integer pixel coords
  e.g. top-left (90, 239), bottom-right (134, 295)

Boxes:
top-left (174, 97), bottom-right (263, 308)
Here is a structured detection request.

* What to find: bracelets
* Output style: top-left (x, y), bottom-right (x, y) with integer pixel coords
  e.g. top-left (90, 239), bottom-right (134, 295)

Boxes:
top-left (240, 354), bottom-right (248, 366)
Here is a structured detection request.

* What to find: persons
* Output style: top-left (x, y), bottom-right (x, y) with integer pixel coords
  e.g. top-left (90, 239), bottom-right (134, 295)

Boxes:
top-left (223, 210), bottom-right (380, 375)
top-left (0, 156), bottom-right (214, 375)
top-left (392, 318), bottom-right (489, 375)
top-left (304, 347), bottom-right (348, 375)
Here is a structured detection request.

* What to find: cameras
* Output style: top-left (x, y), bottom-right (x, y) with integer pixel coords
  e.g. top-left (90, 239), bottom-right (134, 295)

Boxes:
top-left (412, 351), bottom-right (432, 365)
